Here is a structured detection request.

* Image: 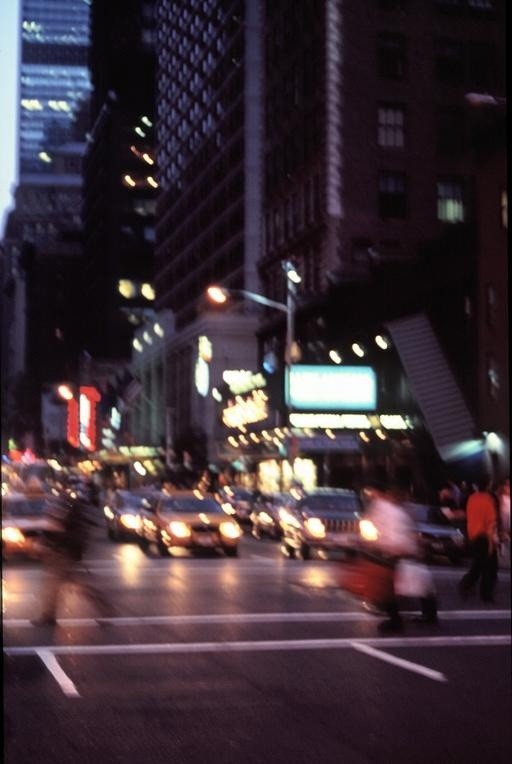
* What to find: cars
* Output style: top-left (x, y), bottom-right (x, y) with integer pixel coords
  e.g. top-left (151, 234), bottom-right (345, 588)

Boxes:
top-left (136, 492), bottom-right (241, 555)
top-left (18, 464), bottom-right (99, 509)
top-left (105, 487), bottom-right (142, 539)
top-left (397, 501), bottom-right (466, 565)
top-left (218, 486), bottom-right (279, 537)
top-left (2, 491), bottom-right (69, 564)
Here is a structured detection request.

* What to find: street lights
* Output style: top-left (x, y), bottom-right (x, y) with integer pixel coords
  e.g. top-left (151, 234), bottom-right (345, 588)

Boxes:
top-left (207, 264), bottom-right (292, 487)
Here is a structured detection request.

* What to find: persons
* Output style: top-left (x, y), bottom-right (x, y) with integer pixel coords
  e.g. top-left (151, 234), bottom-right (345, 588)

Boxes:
top-left (358, 485), bottom-right (438, 632)
top-left (428, 474), bottom-right (512, 594)
top-left (28, 472), bottom-right (119, 628)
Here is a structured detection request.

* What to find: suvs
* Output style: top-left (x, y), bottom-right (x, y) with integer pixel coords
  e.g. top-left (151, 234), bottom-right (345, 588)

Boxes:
top-left (276, 487), bottom-right (371, 561)
